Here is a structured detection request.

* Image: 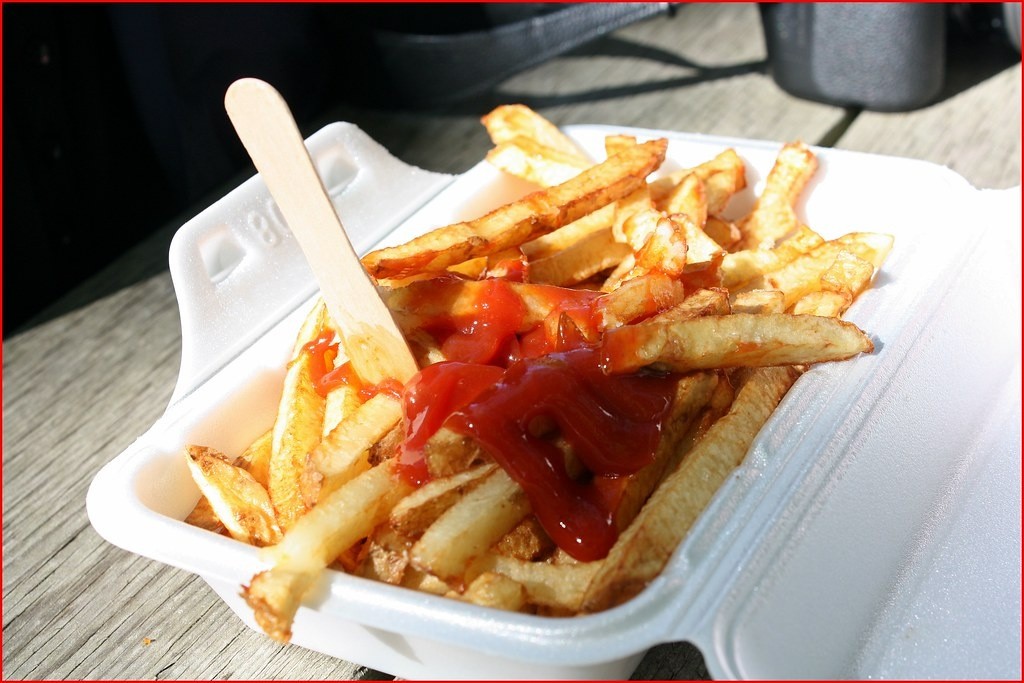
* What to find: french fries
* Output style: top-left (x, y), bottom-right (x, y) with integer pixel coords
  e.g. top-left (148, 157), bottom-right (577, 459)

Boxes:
top-left (184, 105), bottom-right (895, 643)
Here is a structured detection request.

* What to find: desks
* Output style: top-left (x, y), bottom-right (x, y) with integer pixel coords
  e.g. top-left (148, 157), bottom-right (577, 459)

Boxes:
top-left (2, 2), bottom-right (1022, 681)
